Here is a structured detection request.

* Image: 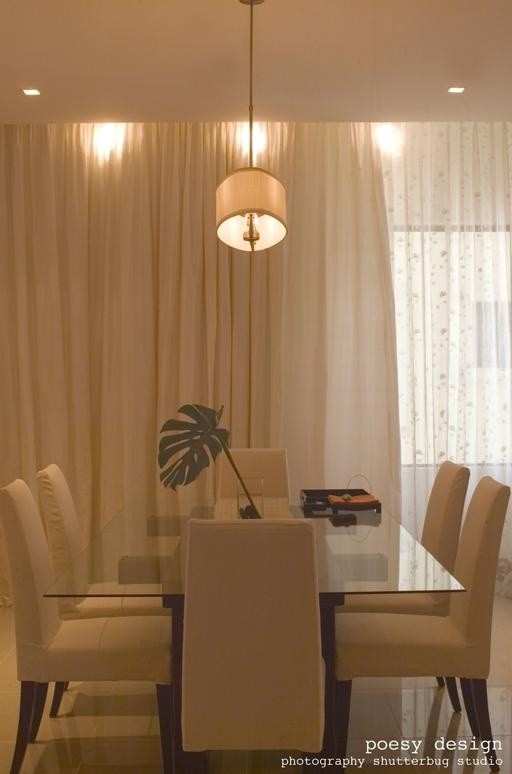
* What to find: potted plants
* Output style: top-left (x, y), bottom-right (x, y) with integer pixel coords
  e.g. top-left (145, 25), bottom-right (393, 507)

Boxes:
top-left (158, 404), bottom-right (262, 519)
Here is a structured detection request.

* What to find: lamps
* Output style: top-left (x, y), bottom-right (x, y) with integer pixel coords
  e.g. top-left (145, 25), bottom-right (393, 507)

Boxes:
top-left (215, 168), bottom-right (287, 253)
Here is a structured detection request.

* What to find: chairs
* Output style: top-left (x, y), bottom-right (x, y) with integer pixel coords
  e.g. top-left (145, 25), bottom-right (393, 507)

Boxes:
top-left (334, 461), bottom-right (471, 712)
top-left (175, 518), bottom-right (326, 774)
top-left (216, 449), bottom-right (294, 519)
top-left (38, 465), bottom-right (173, 718)
top-left (337, 475), bottom-right (511, 768)
top-left (1, 478), bottom-right (171, 774)
